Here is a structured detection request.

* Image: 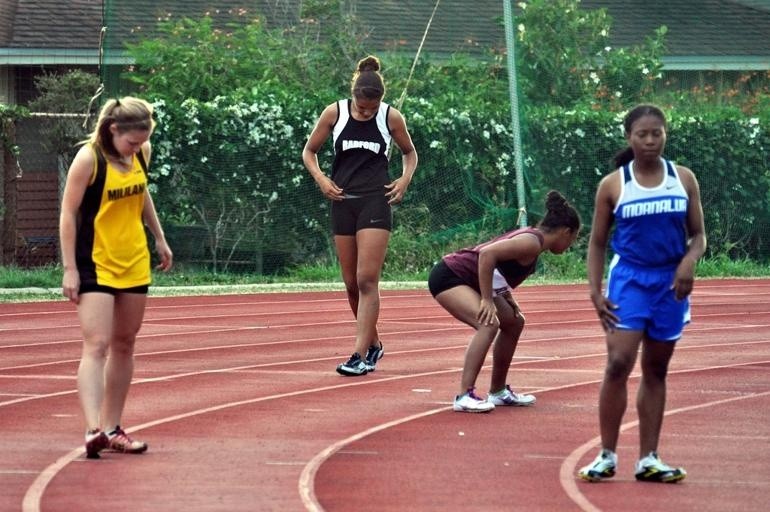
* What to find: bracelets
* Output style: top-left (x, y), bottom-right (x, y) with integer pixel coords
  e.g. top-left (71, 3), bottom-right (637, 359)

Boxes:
top-left (686, 254), bottom-right (698, 265)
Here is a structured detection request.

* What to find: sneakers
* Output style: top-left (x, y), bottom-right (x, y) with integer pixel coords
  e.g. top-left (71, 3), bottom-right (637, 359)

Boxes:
top-left (485, 383), bottom-right (537, 407)
top-left (454, 386), bottom-right (496, 414)
top-left (335, 352), bottom-right (369, 375)
top-left (632, 451), bottom-right (688, 484)
top-left (101, 424), bottom-right (148, 454)
top-left (576, 448), bottom-right (619, 483)
top-left (363, 339), bottom-right (385, 371)
top-left (84, 426), bottom-right (109, 457)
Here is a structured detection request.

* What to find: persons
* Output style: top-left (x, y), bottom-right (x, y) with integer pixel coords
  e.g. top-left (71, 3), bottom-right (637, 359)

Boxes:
top-left (429, 189), bottom-right (582, 416)
top-left (302, 56), bottom-right (419, 377)
top-left (578, 104), bottom-right (709, 484)
top-left (56, 95), bottom-right (174, 458)
